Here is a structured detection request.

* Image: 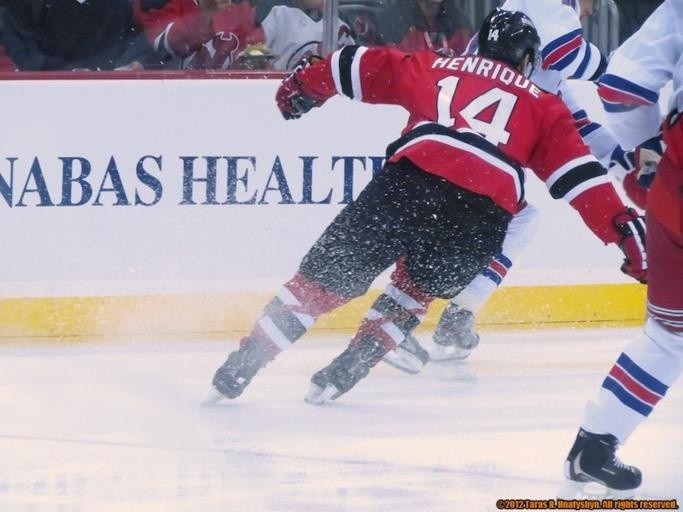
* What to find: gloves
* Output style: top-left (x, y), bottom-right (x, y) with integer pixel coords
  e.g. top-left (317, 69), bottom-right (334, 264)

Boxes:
top-left (276, 53), bottom-right (322, 120)
top-left (609, 139), bottom-right (661, 187)
top-left (612, 207), bottom-right (648, 284)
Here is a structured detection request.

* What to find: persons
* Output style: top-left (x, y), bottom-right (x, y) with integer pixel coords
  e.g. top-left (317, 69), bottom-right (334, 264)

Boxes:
top-left (0, 0), bottom-right (683, 491)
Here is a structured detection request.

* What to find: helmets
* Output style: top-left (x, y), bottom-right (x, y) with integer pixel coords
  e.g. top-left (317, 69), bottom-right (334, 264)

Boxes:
top-left (478, 9), bottom-right (543, 84)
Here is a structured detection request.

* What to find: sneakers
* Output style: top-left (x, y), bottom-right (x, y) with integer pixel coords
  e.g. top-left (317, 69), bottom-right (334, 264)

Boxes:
top-left (310, 338), bottom-right (385, 396)
top-left (564, 428), bottom-right (642, 489)
top-left (434, 302), bottom-right (479, 348)
top-left (213, 339), bottom-right (267, 398)
top-left (400, 334), bottom-right (428, 365)
